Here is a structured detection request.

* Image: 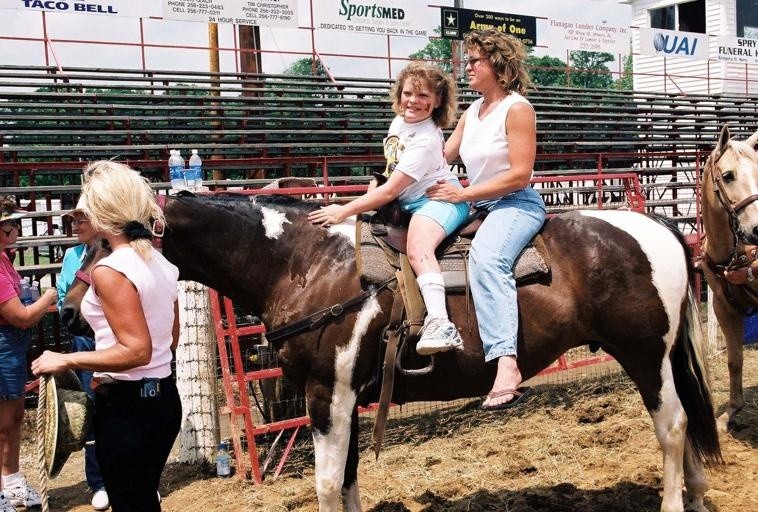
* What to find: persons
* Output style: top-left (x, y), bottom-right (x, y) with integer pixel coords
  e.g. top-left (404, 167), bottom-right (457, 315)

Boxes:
top-left (30, 163), bottom-right (182, 512)
top-left (445, 29), bottom-right (547, 409)
top-left (55, 197), bottom-right (161, 510)
top-left (0, 194), bottom-right (59, 512)
top-left (307, 64), bottom-right (471, 356)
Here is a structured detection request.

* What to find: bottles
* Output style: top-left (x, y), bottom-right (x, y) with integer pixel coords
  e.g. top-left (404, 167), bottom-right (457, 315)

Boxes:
top-left (168, 147), bottom-right (203, 192)
top-left (19, 277), bottom-right (40, 307)
top-left (216, 443), bottom-right (231, 478)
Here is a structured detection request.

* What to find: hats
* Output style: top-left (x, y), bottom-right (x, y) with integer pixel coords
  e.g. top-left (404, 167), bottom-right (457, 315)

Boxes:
top-left (59, 191), bottom-right (84, 218)
top-left (0, 208), bottom-right (29, 223)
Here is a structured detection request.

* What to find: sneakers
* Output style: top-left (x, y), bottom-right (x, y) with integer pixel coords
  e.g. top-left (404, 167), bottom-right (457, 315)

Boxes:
top-left (0, 476), bottom-right (42, 512)
top-left (91, 489), bottom-right (110, 511)
top-left (156, 489), bottom-right (161, 504)
top-left (414, 316), bottom-right (466, 356)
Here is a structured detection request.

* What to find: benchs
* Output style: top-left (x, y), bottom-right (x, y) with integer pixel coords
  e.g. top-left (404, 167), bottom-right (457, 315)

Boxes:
top-left (0, 62), bottom-right (758, 388)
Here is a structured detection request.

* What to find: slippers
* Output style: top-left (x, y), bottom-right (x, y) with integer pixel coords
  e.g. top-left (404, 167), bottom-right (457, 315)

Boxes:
top-left (478, 385), bottom-right (533, 410)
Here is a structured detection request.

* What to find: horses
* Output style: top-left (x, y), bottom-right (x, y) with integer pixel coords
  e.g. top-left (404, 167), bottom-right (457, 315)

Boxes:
top-left (58, 189), bottom-right (730, 512)
top-left (697, 119), bottom-right (757, 430)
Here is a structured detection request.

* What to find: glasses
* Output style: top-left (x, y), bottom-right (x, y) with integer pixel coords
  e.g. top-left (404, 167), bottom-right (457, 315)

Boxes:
top-left (70, 217), bottom-right (91, 229)
top-left (462, 54), bottom-right (489, 67)
top-left (0, 220), bottom-right (19, 227)
